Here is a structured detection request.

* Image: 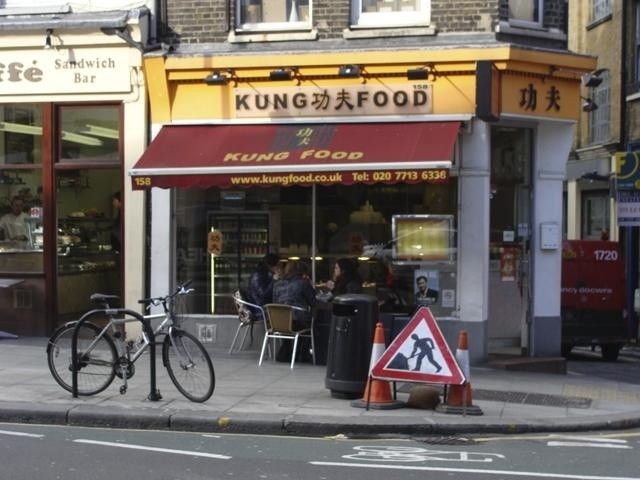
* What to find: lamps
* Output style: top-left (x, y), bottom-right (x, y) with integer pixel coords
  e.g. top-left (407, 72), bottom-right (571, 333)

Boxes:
top-left (269, 65), bottom-right (298, 81)
top-left (43, 28), bottom-right (55, 49)
top-left (406, 61), bottom-right (432, 81)
top-left (337, 64), bottom-right (363, 79)
top-left (203, 68), bottom-right (235, 86)
top-left (579, 95), bottom-right (599, 113)
top-left (583, 72), bottom-right (605, 89)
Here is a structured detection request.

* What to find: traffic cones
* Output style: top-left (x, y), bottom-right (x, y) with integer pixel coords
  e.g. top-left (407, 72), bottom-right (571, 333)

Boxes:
top-left (434, 331), bottom-right (483, 416)
top-left (351, 322), bottom-right (404, 410)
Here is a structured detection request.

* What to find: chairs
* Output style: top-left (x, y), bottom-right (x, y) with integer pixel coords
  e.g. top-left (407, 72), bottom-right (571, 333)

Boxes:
top-left (226, 294), bottom-right (284, 360)
top-left (257, 302), bottom-right (318, 370)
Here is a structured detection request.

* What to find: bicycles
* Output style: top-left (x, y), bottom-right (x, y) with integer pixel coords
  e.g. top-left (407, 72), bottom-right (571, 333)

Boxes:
top-left (45, 278), bottom-right (215, 402)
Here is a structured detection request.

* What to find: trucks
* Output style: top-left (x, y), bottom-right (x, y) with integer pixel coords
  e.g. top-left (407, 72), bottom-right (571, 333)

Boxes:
top-left (561, 241), bottom-right (628, 362)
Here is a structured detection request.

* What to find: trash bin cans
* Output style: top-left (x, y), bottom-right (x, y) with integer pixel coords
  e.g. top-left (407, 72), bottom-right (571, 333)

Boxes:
top-left (325, 293), bottom-right (377, 400)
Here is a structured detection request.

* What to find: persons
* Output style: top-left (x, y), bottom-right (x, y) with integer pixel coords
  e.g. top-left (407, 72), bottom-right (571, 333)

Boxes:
top-left (308, 257), bottom-right (361, 366)
top-left (89, 190), bottom-right (122, 255)
top-left (245, 252), bottom-right (284, 321)
top-left (21, 205), bottom-right (32, 217)
top-left (271, 259), bottom-right (324, 367)
top-left (0, 194), bottom-right (34, 241)
top-left (414, 274), bottom-right (439, 303)
